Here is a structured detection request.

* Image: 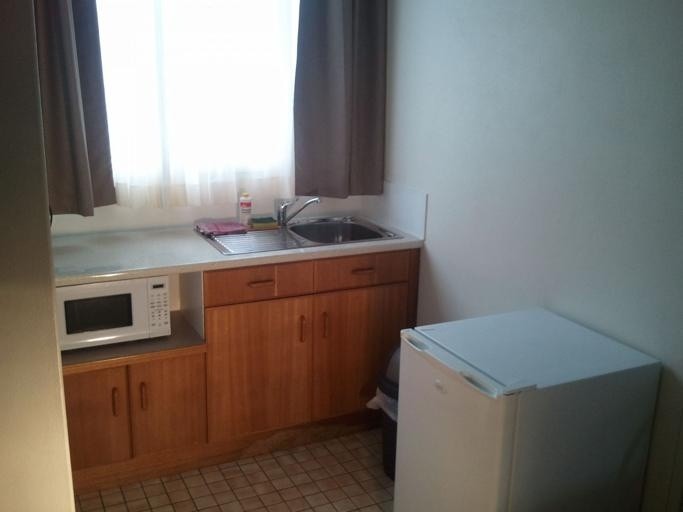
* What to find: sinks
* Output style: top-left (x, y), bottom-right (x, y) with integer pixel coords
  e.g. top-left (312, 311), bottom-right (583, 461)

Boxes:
top-left (290, 221), bottom-right (385, 244)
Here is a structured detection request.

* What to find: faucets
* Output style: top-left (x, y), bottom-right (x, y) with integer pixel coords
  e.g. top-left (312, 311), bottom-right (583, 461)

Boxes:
top-left (276, 197), bottom-right (321, 226)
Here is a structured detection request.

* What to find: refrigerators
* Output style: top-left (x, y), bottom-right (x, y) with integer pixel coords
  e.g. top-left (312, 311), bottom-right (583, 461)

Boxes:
top-left (393, 302), bottom-right (661, 512)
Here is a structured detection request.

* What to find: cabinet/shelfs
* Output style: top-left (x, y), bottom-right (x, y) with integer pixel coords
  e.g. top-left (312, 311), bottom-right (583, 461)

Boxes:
top-left (205, 250), bottom-right (417, 440)
top-left (62, 311), bottom-right (207, 471)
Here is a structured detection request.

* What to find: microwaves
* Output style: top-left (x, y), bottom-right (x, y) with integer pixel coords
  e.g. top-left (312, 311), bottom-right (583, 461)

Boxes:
top-left (55, 272), bottom-right (173, 351)
